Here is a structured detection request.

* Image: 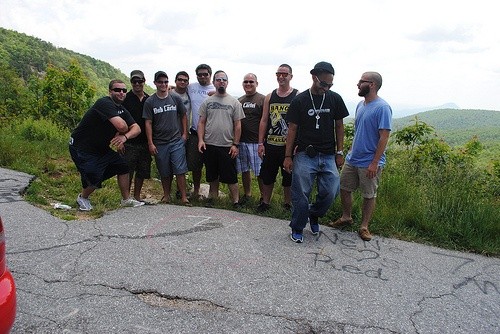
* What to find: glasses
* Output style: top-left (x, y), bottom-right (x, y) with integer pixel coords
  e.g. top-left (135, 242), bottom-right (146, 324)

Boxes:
top-left (214, 78), bottom-right (227, 82)
top-left (110, 88), bottom-right (127, 93)
top-left (359, 79), bottom-right (372, 84)
top-left (315, 76), bottom-right (333, 88)
top-left (131, 79), bottom-right (144, 84)
top-left (276, 71), bottom-right (292, 77)
top-left (177, 78), bottom-right (189, 83)
top-left (243, 80), bottom-right (255, 84)
top-left (197, 72), bottom-right (210, 78)
top-left (155, 80), bottom-right (168, 84)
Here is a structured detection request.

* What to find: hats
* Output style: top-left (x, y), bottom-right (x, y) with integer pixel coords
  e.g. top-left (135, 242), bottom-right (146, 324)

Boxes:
top-left (154, 71), bottom-right (168, 80)
top-left (130, 70), bottom-right (144, 82)
top-left (310, 62), bottom-right (335, 75)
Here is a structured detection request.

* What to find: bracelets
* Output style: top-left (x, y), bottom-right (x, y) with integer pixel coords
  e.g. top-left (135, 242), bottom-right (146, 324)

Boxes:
top-left (286, 155), bottom-right (291, 158)
top-left (258, 141), bottom-right (264, 146)
top-left (123, 134), bottom-right (128, 140)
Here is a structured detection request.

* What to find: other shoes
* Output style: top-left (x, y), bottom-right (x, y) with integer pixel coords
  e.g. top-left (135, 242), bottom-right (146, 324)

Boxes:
top-left (232, 201), bottom-right (239, 209)
top-left (181, 197), bottom-right (193, 207)
top-left (241, 194), bottom-right (253, 203)
top-left (283, 203), bottom-right (292, 212)
top-left (160, 196), bottom-right (172, 204)
top-left (255, 203), bottom-right (271, 213)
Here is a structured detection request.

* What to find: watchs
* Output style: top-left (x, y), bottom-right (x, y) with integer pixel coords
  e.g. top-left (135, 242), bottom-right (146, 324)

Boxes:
top-left (336, 150), bottom-right (343, 156)
top-left (233, 143), bottom-right (240, 148)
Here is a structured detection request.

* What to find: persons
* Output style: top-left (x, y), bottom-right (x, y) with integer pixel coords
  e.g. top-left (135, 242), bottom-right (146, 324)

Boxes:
top-left (257, 64), bottom-right (300, 210)
top-left (235, 72), bottom-right (266, 201)
top-left (283, 62), bottom-right (350, 244)
top-left (197, 71), bottom-right (246, 205)
top-left (167, 64), bottom-right (215, 199)
top-left (168, 71), bottom-right (192, 170)
top-left (68, 80), bottom-right (145, 211)
top-left (329, 72), bottom-right (393, 240)
top-left (123, 71), bottom-right (151, 203)
top-left (143, 71), bottom-right (191, 206)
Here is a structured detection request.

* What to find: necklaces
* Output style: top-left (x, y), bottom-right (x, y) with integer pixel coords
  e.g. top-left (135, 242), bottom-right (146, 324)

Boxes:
top-left (309, 88), bottom-right (325, 129)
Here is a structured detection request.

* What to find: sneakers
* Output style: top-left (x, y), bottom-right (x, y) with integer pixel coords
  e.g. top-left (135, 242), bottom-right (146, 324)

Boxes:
top-left (121, 197), bottom-right (145, 207)
top-left (308, 204), bottom-right (320, 234)
top-left (291, 229), bottom-right (304, 243)
top-left (328, 216), bottom-right (354, 227)
top-left (358, 226), bottom-right (372, 241)
top-left (76, 193), bottom-right (94, 212)
